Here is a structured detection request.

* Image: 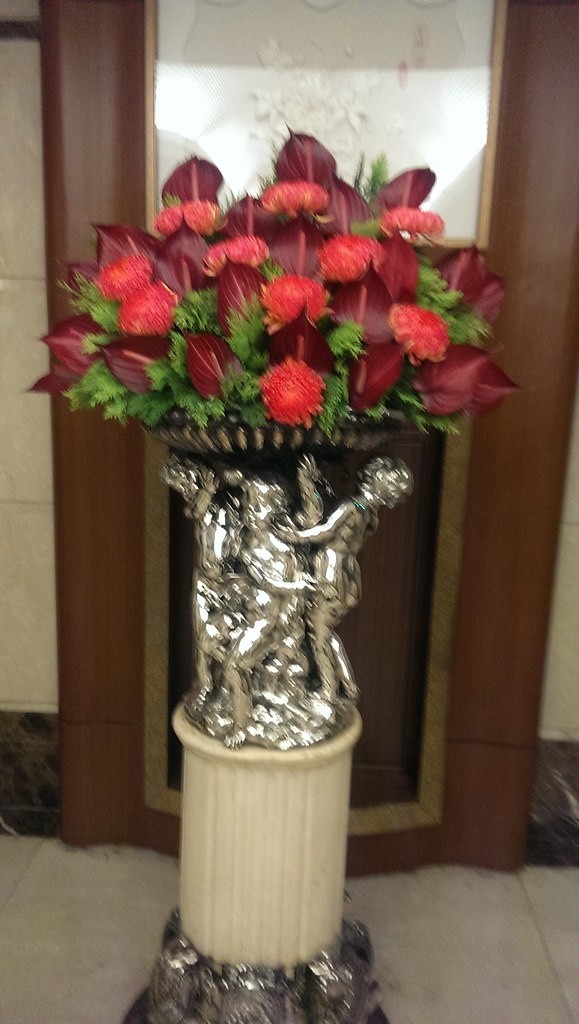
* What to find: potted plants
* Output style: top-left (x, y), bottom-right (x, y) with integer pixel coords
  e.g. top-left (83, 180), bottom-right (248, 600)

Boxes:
top-left (4, 124), bottom-right (522, 1024)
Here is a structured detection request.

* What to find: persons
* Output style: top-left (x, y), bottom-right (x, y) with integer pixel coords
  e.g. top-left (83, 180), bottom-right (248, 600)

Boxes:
top-left (151, 449), bottom-right (416, 744)
